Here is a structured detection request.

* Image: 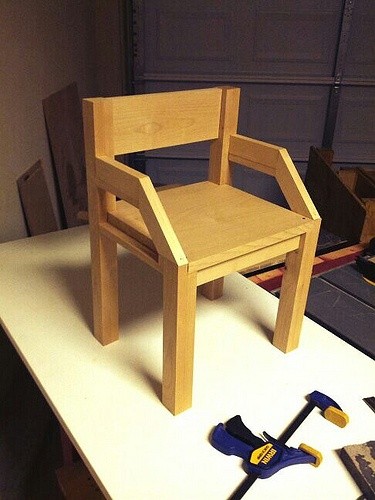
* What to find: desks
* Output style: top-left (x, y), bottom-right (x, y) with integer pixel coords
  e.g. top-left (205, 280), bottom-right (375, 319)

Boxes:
top-left (1, 222), bottom-right (375, 498)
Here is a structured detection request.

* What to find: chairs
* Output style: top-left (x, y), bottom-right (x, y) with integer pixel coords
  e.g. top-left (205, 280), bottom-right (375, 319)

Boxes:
top-left (82, 85), bottom-right (323, 415)
